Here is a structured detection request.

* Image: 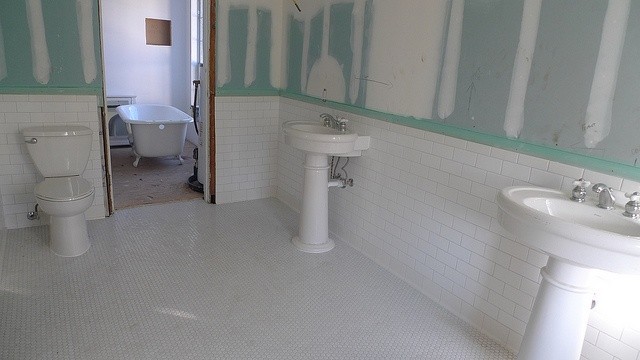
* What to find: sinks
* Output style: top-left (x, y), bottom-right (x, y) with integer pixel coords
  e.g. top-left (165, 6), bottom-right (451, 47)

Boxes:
top-left (524, 197), bottom-right (640, 237)
top-left (292, 125), bottom-right (341, 134)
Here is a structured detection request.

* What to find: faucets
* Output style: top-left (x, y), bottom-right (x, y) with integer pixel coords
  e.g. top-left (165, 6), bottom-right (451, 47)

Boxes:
top-left (320, 113), bottom-right (348, 131)
top-left (570, 178), bottom-right (640, 217)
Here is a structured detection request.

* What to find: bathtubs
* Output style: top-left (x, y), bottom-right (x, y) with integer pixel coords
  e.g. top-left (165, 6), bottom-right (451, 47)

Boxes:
top-left (124, 105), bottom-right (190, 120)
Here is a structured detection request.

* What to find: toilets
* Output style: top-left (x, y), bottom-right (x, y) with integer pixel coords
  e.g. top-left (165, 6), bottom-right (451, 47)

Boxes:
top-left (22, 125), bottom-right (95, 257)
